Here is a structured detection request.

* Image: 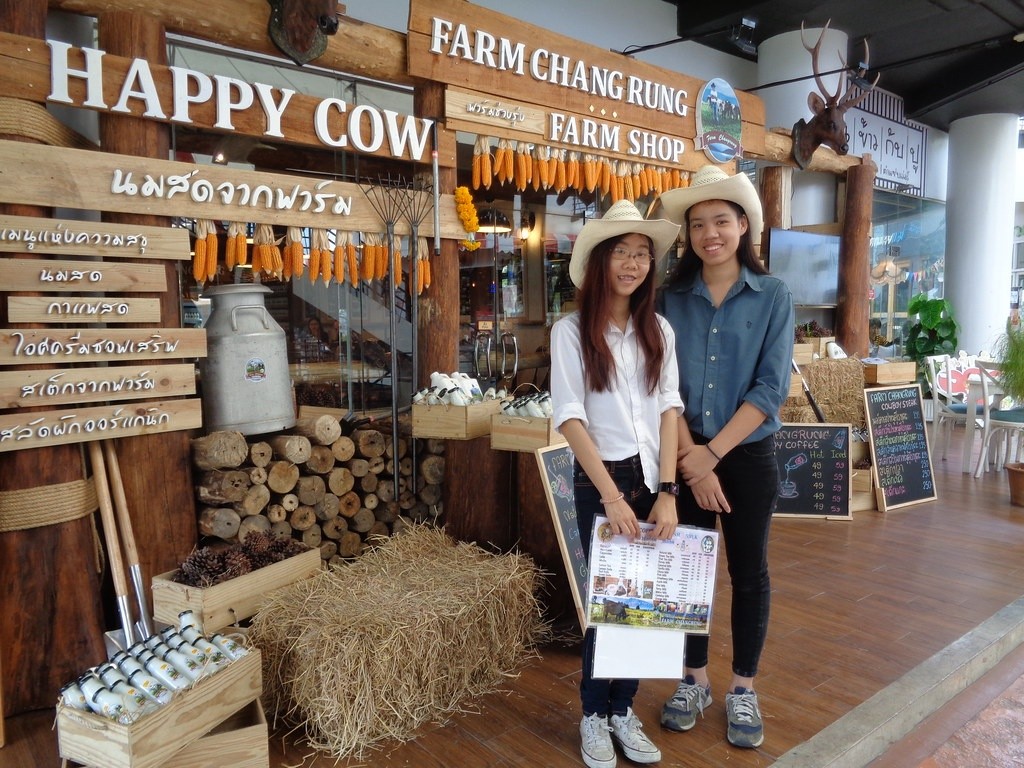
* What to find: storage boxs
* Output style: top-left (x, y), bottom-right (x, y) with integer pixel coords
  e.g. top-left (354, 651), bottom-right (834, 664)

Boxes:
top-left (55, 545), bottom-right (320, 768)
top-left (412, 396), bottom-right (570, 454)
top-left (786, 337), bottom-right (916, 513)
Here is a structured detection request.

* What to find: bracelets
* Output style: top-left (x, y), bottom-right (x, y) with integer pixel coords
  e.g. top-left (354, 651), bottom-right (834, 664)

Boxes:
top-left (600, 492), bottom-right (624, 503)
top-left (706, 443), bottom-right (721, 461)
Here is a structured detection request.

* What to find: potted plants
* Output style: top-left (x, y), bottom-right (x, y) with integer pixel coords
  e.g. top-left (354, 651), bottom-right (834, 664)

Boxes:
top-left (904, 293), bottom-right (957, 425)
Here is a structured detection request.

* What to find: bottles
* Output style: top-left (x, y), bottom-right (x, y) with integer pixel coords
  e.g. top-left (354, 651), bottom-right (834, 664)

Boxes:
top-left (812, 353), bottom-right (819, 360)
top-left (411, 372), bottom-right (553, 418)
top-left (60, 610), bottom-right (251, 723)
top-left (826, 341), bottom-right (848, 359)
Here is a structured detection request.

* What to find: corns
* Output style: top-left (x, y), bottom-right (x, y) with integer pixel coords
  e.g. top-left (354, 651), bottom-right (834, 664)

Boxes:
top-left (192, 234), bottom-right (432, 297)
top-left (472, 148), bottom-right (692, 205)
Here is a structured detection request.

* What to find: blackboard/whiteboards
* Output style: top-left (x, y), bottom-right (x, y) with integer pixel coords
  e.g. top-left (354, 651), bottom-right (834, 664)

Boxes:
top-left (535, 442), bottom-right (588, 636)
top-left (774, 423), bottom-right (853, 520)
top-left (863, 383), bottom-right (938, 512)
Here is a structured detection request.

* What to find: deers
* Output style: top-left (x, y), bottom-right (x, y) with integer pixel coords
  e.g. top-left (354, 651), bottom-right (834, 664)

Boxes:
top-left (795, 16), bottom-right (880, 161)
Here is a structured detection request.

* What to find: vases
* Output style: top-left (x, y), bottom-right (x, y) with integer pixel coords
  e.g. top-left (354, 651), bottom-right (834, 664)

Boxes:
top-left (1004, 463), bottom-right (1024, 508)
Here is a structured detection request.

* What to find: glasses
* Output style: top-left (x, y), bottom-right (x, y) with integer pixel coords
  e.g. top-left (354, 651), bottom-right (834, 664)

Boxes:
top-left (610, 248), bottom-right (655, 264)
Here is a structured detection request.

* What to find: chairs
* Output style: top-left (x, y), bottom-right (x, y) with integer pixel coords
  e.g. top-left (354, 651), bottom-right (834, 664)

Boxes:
top-left (921, 349), bottom-right (1024, 479)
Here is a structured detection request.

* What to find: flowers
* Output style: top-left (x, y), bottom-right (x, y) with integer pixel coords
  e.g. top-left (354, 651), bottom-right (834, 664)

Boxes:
top-left (454, 186), bottom-right (481, 252)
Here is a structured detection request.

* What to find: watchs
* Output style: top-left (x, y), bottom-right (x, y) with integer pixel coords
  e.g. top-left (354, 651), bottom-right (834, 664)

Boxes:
top-left (658, 482), bottom-right (679, 495)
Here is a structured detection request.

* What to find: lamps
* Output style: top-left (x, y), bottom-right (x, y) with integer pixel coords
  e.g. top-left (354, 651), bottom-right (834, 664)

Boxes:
top-left (521, 220), bottom-right (530, 242)
top-left (475, 190), bottom-right (512, 233)
top-left (621, 14), bottom-right (760, 65)
top-left (870, 215), bottom-right (910, 286)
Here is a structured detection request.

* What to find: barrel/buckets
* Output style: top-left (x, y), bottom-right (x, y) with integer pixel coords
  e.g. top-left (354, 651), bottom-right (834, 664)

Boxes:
top-left (199, 284), bottom-right (297, 436)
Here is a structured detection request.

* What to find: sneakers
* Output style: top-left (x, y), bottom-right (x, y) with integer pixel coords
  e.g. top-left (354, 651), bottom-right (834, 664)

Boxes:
top-left (608, 706), bottom-right (661, 763)
top-left (660, 673), bottom-right (712, 730)
top-left (724, 686), bottom-right (764, 747)
top-left (579, 712), bottom-right (617, 768)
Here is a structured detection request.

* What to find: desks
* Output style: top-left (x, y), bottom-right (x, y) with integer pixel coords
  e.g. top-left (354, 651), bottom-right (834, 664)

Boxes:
top-left (961, 373), bottom-right (1014, 475)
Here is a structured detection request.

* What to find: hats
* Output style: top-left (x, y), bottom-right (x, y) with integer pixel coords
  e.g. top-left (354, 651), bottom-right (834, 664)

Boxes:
top-left (569, 199), bottom-right (681, 290)
top-left (659, 165), bottom-right (763, 245)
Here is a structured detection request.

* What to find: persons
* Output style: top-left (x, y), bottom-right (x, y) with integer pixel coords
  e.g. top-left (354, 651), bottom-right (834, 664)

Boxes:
top-left (304, 317), bottom-right (329, 343)
top-left (710, 83), bottom-right (719, 124)
top-left (550, 199), bottom-right (685, 768)
top-left (653, 164), bottom-right (795, 746)
top-left (869, 319), bottom-right (900, 359)
